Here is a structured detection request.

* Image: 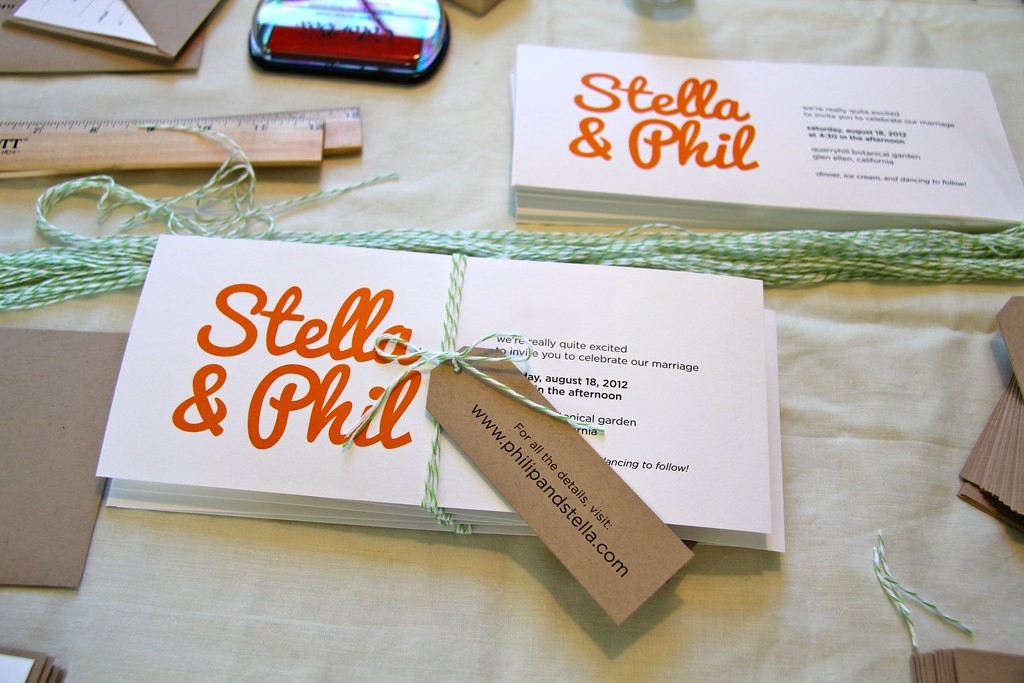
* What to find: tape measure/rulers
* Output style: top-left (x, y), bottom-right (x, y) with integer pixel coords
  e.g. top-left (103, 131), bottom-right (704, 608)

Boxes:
top-left (0, 104), bottom-right (363, 180)
